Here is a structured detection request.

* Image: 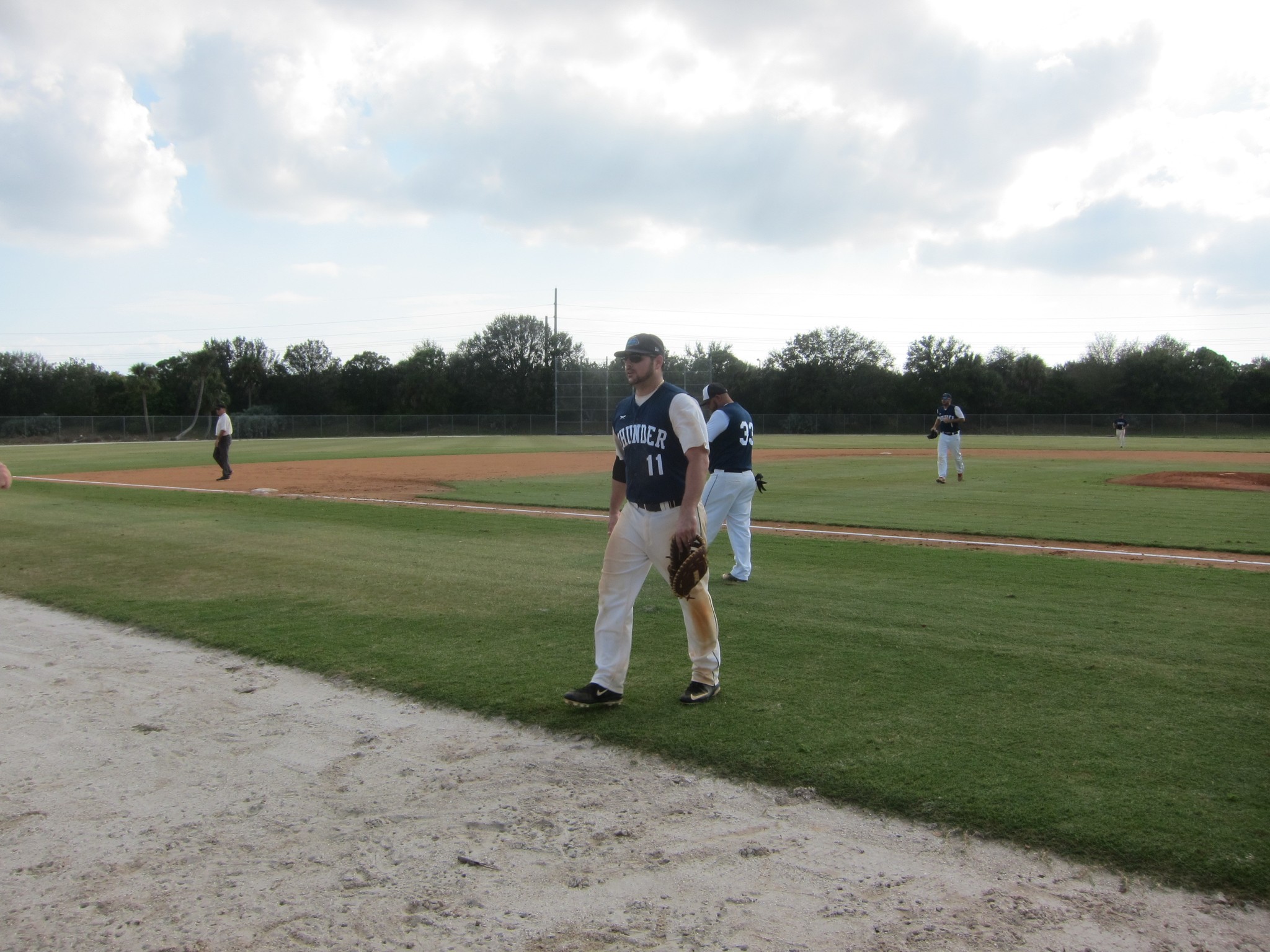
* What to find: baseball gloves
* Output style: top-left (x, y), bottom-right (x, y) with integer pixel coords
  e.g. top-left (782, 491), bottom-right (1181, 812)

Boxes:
top-left (927, 430), bottom-right (939, 439)
top-left (668, 532), bottom-right (709, 600)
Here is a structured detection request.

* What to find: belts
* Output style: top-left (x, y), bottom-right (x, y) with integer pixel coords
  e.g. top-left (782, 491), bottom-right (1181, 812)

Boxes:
top-left (941, 431), bottom-right (958, 435)
top-left (711, 469), bottom-right (750, 473)
top-left (638, 499), bottom-right (682, 512)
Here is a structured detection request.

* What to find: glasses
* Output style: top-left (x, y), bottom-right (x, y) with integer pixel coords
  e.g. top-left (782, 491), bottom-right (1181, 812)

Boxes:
top-left (215, 408), bottom-right (222, 410)
top-left (621, 354), bottom-right (657, 364)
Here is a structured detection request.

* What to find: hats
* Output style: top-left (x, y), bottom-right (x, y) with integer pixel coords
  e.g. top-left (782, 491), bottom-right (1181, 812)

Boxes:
top-left (215, 403), bottom-right (227, 410)
top-left (614, 333), bottom-right (664, 360)
top-left (941, 393), bottom-right (952, 399)
top-left (699, 383), bottom-right (728, 407)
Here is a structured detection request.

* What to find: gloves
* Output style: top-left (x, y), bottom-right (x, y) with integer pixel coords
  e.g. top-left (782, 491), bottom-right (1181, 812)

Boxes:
top-left (755, 473), bottom-right (768, 493)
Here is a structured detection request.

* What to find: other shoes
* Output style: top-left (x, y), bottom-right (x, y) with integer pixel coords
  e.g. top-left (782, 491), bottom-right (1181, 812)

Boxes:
top-left (721, 573), bottom-right (748, 582)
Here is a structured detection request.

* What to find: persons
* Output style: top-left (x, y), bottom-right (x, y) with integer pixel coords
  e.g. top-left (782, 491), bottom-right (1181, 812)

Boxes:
top-left (930, 393), bottom-right (965, 483)
top-left (699, 382), bottom-right (756, 582)
top-left (563, 332), bottom-right (723, 706)
top-left (213, 402), bottom-right (234, 481)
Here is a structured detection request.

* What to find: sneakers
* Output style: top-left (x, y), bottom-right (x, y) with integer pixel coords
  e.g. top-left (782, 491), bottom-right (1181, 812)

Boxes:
top-left (679, 681), bottom-right (720, 704)
top-left (217, 475), bottom-right (230, 481)
top-left (563, 682), bottom-right (623, 707)
top-left (958, 472), bottom-right (963, 482)
top-left (936, 477), bottom-right (946, 483)
top-left (228, 469), bottom-right (234, 476)
